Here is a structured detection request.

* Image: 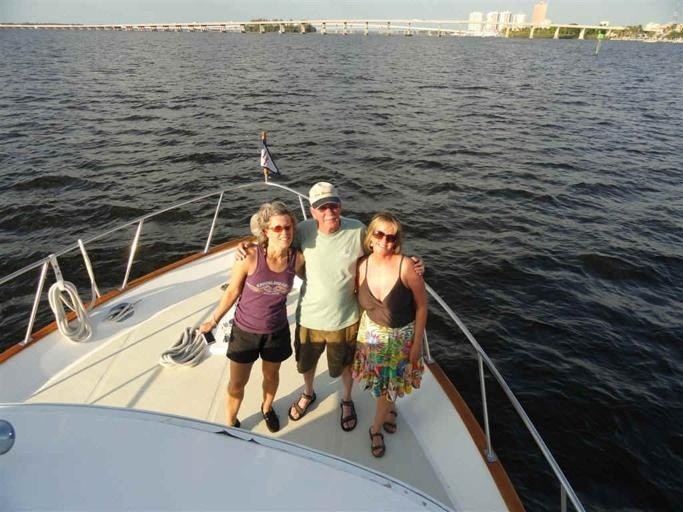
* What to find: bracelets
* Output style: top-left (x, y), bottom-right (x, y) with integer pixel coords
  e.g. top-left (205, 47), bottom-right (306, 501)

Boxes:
top-left (209, 313), bottom-right (219, 329)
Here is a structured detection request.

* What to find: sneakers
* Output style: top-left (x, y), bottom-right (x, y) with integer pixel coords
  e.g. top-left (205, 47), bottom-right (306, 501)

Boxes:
top-left (261, 402), bottom-right (279, 432)
top-left (235, 418), bottom-right (240, 427)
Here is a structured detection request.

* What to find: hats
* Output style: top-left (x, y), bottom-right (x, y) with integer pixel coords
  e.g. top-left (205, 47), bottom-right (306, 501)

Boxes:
top-left (309, 181), bottom-right (342, 209)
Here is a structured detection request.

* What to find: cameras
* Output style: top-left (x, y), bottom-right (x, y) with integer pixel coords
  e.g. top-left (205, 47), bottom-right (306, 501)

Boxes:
top-left (201, 332), bottom-right (217, 346)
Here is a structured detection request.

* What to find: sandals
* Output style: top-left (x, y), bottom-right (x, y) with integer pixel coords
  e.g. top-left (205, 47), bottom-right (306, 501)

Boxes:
top-left (369, 426), bottom-right (386, 457)
top-left (340, 398), bottom-right (357, 431)
top-left (383, 411), bottom-right (398, 433)
top-left (288, 389), bottom-right (316, 421)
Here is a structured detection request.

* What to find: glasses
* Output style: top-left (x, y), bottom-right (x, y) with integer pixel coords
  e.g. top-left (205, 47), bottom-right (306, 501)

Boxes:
top-left (373, 230), bottom-right (397, 243)
top-left (265, 225), bottom-right (292, 233)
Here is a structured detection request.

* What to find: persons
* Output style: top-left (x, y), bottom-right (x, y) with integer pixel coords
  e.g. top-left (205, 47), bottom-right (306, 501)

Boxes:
top-left (236, 180), bottom-right (426, 431)
top-left (352, 210), bottom-right (428, 459)
top-left (195, 199), bottom-right (303, 433)
